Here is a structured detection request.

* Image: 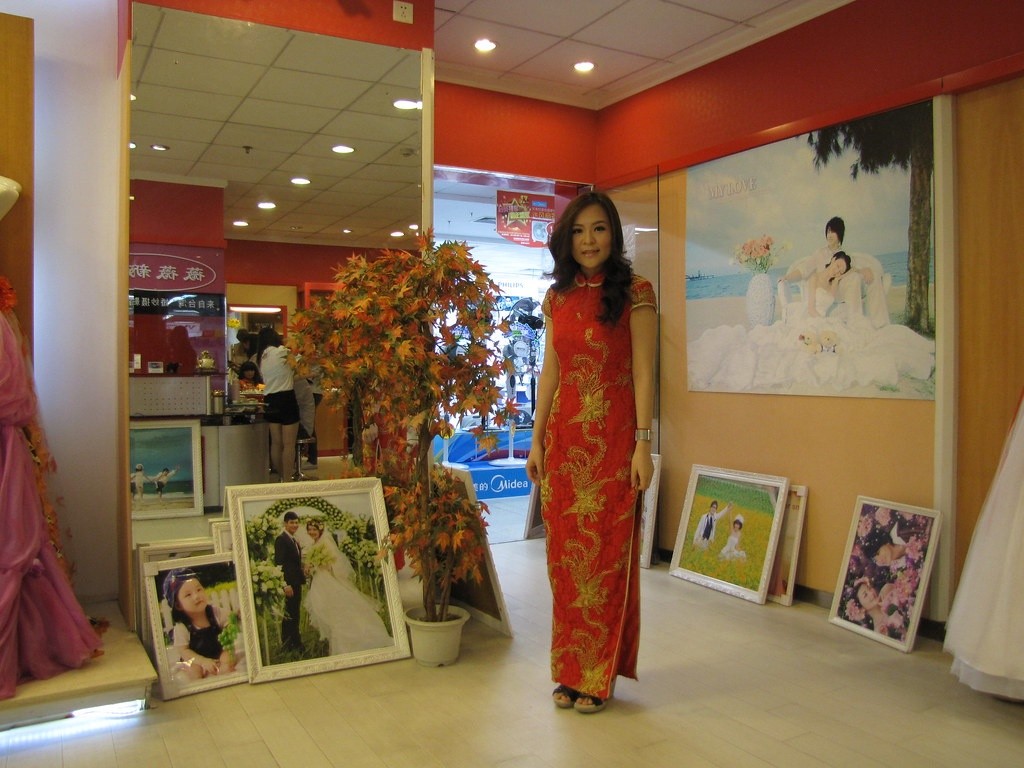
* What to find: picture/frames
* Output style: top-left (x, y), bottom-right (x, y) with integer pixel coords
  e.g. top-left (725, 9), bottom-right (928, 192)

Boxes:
top-left (130, 419), bottom-right (204, 520)
top-left (638, 453), bottom-right (662, 569)
top-left (829, 494), bottom-right (943, 652)
top-left (766, 484), bottom-right (808, 606)
top-left (524, 451), bottom-right (545, 541)
top-left (138, 522), bottom-right (251, 702)
top-left (668, 464), bottom-right (791, 605)
top-left (226, 476), bottom-right (412, 685)
top-left (434, 461), bottom-right (515, 639)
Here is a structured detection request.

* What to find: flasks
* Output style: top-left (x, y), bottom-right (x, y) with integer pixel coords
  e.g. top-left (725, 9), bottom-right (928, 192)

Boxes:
top-left (212, 389), bottom-right (224, 415)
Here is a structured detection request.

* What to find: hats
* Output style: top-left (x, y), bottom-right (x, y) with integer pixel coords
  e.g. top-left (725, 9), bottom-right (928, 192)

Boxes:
top-left (136, 464), bottom-right (144, 470)
top-left (163, 568), bottom-right (200, 612)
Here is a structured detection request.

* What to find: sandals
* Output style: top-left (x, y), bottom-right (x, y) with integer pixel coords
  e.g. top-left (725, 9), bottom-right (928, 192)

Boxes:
top-left (552, 685), bottom-right (579, 707)
top-left (574, 678), bottom-right (614, 712)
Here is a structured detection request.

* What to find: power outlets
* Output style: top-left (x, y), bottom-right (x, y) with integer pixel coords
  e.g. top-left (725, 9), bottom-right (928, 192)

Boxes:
top-left (391, 1), bottom-right (415, 26)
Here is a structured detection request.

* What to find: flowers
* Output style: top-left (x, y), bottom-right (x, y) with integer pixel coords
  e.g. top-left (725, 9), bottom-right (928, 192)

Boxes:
top-left (844, 507), bottom-right (927, 640)
top-left (728, 234), bottom-right (792, 276)
top-left (245, 497), bottom-right (383, 618)
top-left (799, 331), bottom-right (842, 355)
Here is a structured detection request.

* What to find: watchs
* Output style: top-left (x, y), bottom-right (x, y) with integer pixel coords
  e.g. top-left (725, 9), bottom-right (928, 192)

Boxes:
top-left (635, 428), bottom-right (654, 442)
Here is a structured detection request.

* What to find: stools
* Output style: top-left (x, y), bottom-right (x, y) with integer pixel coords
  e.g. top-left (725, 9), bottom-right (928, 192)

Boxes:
top-left (292, 433), bottom-right (320, 481)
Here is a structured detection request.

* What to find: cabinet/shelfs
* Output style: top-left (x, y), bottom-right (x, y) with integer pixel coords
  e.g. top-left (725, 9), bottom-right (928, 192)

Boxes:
top-left (434, 425), bottom-right (536, 500)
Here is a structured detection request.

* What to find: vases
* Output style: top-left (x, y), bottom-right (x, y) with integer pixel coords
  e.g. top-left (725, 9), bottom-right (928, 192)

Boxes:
top-left (744, 273), bottom-right (777, 326)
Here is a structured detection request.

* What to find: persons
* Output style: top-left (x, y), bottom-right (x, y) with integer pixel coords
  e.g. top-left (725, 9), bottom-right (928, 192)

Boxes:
top-left (285, 343), bottom-right (324, 470)
top-left (162, 568), bottom-right (238, 684)
top-left (716, 503), bottom-right (749, 562)
top-left (257, 327), bottom-right (299, 483)
top-left (127, 307), bottom-right (199, 380)
top-left (693, 499), bottom-right (732, 549)
top-left (127, 462), bottom-right (180, 502)
top-left (779, 214), bottom-right (890, 396)
top-left (273, 510), bottom-right (392, 657)
top-left (523, 194), bottom-right (659, 715)
top-left (236, 364), bottom-right (262, 389)
top-left (267, 348), bottom-right (315, 475)
top-left (230, 327), bottom-right (260, 375)
top-left (852, 522), bottom-right (928, 641)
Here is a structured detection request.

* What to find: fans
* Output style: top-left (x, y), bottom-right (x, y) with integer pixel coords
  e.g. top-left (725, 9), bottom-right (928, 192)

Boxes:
top-left (435, 293), bottom-right (547, 471)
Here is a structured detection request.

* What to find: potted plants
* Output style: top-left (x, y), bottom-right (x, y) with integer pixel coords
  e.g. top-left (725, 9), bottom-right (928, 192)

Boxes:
top-left (281, 226), bottom-right (526, 668)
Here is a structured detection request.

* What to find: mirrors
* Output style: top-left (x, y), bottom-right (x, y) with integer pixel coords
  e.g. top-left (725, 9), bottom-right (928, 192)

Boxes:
top-left (227, 305), bottom-right (288, 384)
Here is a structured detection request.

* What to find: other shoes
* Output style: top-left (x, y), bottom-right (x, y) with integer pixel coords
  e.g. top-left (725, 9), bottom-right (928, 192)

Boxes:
top-left (302, 460), bottom-right (318, 469)
